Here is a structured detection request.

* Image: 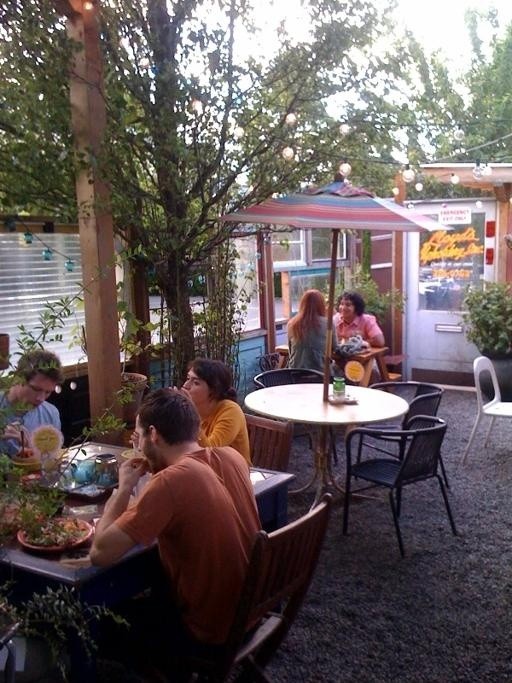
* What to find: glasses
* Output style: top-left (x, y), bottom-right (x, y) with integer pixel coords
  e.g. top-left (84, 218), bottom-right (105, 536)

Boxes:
top-left (24, 378), bottom-right (55, 397)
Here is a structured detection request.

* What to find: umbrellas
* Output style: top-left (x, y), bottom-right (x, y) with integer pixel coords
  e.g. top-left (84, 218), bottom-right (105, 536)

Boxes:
top-left (219, 167), bottom-right (453, 403)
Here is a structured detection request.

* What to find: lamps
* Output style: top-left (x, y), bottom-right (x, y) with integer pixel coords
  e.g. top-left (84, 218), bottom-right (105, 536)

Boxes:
top-left (416, 182), bottom-right (425, 191)
top-left (471, 163), bottom-right (482, 178)
top-left (393, 188), bottom-right (399, 194)
top-left (22, 233), bottom-right (31, 242)
top-left (281, 147), bottom-right (294, 163)
top-left (474, 198), bottom-right (479, 206)
top-left (339, 123), bottom-right (347, 133)
top-left (64, 260), bottom-right (72, 269)
top-left (338, 161), bottom-right (350, 174)
top-left (453, 128), bottom-right (464, 137)
top-left (402, 169), bottom-right (414, 180)
top-left (285, 111), bottom-right (295, 124)
top-left (42, 246), bottom-right (52, 259)
top-left (451, 176), bottom-right (461, 183)
top-left (186, 98), bottom-right (200, 117)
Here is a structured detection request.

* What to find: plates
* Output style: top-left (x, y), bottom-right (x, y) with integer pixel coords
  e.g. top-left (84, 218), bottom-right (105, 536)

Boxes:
top-left (17, 516), bottom-right (94, 552)
top-left (46, 480), bottom-right (119, 504)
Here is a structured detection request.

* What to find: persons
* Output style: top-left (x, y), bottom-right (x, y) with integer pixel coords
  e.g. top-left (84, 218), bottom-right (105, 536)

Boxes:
top-left (129, 356), bottom-right (251, 467)
top-left (77, 387), bottom-right (262, 682)
top-left (0, 349), bottom-right (61, 458)
top-left (333, 291), bottom-right (394, 386)
top-left (286, 288), bottom-right (346, 384)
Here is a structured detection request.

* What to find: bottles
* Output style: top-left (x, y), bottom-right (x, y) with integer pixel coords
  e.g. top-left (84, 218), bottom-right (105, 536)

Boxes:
top-left (333, 377), bottom-right (345, 399)
top-left (94, 455), bottom-right (119, 489)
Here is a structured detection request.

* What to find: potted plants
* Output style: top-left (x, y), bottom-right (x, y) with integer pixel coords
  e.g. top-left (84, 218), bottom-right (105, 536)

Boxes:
top-left (459, 281), bottom-right (512, 404)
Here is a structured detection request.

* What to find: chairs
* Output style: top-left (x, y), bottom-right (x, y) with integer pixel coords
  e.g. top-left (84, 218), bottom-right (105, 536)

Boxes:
top-left (253, 369), bottom-right (334, 389)
top-left (460, 356), bottom-right (512, 464)
top-left (343, 415), bottom-right (462, 552)
top-left (212, 493), bottom-right (337, 683)
top-left (246, 412), bottom-right (293, 469)
top-left (355, 381), bottom-right (452, 493)
